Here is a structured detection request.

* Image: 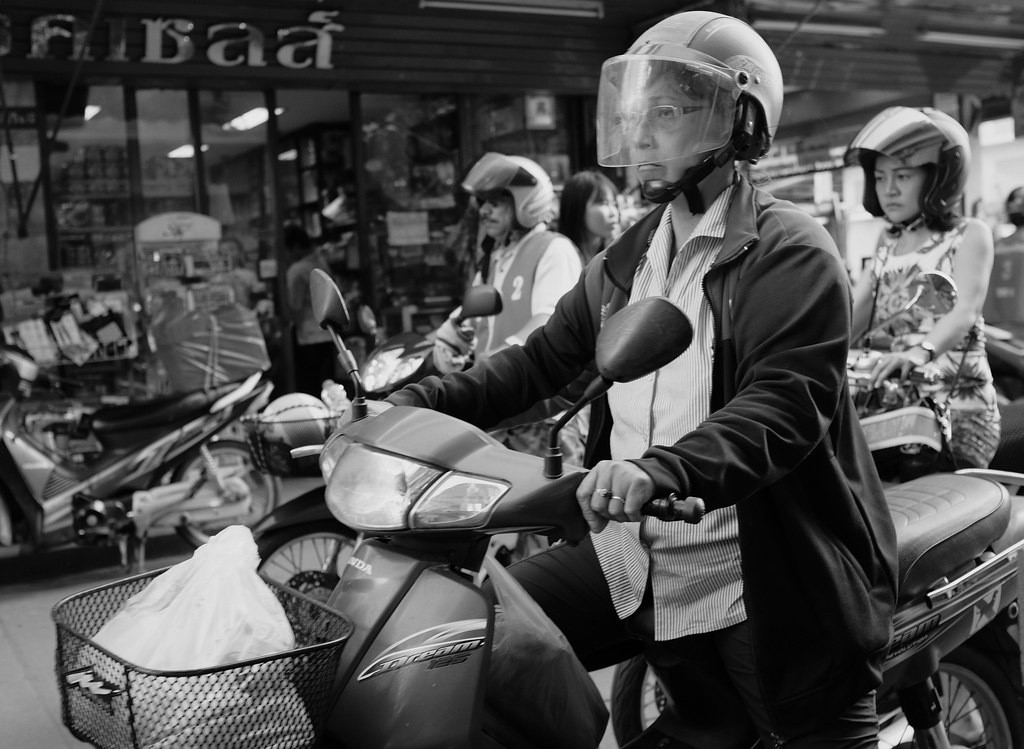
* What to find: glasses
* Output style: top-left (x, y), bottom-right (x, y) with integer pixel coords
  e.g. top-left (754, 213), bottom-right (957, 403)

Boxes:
top-left (609, 104), bottom-right (717, 136)
top-left (475, 192), bottom-right (511, 207)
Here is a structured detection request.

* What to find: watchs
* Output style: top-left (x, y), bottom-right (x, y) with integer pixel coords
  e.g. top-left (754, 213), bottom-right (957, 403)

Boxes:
top-left (904, 340), bottom-right (937, 361)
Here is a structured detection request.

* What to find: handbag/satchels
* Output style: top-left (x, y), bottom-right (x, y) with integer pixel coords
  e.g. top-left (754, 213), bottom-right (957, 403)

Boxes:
top-left (876, 395), bottom-right (960, 484)
top-left (469, 554), bottom-right (611, 749)
top-left (65, 525), bottom-right (315, 749)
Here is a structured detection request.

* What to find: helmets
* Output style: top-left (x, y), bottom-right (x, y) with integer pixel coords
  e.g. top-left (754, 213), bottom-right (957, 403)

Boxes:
top-left (603, 10), bottom-right (784, 160)
top-left (858, 108), bottom-right (971, 216)
top-left (480, 155), bottom-right (553, 228)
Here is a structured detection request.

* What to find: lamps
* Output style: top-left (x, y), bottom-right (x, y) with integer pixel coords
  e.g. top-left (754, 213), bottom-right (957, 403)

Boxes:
top-left (744, 0), bottom-right (1024, 48)
top-left (418, 0), bottom-right (606, 19)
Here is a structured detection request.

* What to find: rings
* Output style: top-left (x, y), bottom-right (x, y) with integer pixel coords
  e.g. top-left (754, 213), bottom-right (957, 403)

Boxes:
top-left (593, 488), bottom-right (613, 497)
top-left (877, 358), bottom-right (883, 363)
top-left (612, 495), bottom-right (624, 504)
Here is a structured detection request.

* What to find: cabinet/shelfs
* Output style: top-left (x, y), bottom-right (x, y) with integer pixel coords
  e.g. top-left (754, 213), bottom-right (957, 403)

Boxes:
top-left (248, 126), bottom-right (354, 245)
top-left (407, 109), bottom-right (465, 195)
top-left (53, 195), bottom-right (133, 234)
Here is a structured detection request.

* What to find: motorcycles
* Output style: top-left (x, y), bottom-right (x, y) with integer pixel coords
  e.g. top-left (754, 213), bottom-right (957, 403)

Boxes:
top-left (0, 281), bottom-right (1023, 574)
top-left (46, 266), bottom-right (1023, 747)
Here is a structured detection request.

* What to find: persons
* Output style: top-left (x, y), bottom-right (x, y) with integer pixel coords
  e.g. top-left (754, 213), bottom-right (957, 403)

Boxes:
top-left (255, 237), bottom-right (293, 314)
top-left (217, 238), bottom-right (260, 295)
top-left (837, 106), bottom-right (1003, 478)
top-left (327, 8), bottom-right (901, 749)
top-left (559, 172), bottom-right (625, 268)
top-left (983, 186), bottom-right (1024, 355)
top-left (462, 151), bottom-right (587, 469)
top-left (161, 255), bottom-right (184, 277)
top-left (271, 222), bottom-right (342, 395)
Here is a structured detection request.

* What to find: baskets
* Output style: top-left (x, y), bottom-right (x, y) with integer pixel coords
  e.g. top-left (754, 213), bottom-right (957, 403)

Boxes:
top-left (239, 409), bottom-right (341, 479)
top-left (51, 561), bottom-right (356, 749)
